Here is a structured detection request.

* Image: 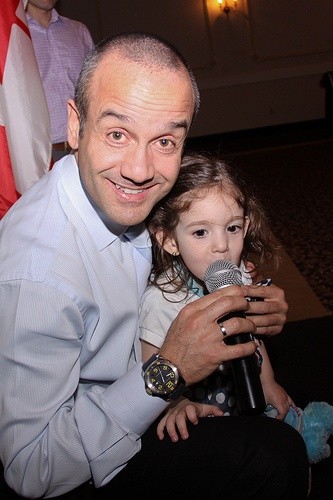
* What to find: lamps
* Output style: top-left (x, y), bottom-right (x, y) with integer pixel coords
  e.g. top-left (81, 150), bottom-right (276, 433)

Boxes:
top-left (216, 0), bottom-right (238, 26)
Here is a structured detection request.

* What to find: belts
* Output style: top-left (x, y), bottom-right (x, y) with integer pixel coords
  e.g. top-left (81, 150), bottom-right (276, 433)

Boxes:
top-left (52, 140), bottom-right (72, 151)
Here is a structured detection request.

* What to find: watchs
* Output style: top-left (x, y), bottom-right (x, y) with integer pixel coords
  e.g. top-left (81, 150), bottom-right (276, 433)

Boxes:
top-left (139, 352), bottom-right (187, 403)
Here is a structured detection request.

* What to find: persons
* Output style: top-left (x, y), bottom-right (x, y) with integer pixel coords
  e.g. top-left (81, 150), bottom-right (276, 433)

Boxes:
top-left (137, 154), bottom-right (299, 446)
top-left (0, 32), bottom-right (314, 500)
top-left (22, 0), bottom-right (98, 168)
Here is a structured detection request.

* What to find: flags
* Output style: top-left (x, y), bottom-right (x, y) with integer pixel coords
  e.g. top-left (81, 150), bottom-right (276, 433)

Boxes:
top-left (1, 0), bottom-right (55, 219)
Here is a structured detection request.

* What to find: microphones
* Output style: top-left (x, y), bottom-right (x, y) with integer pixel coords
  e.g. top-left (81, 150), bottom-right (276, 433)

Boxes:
top-left (203, 259), bottom-right (269, 416)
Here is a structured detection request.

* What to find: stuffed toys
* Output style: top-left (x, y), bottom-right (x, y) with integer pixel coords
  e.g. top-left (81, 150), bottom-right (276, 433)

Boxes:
top-left (264, 400), bottom-right (333, 466)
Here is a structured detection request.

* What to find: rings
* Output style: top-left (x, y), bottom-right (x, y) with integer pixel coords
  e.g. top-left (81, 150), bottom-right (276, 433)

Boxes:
top-left (219, 323), bottom-right (229, 339)
